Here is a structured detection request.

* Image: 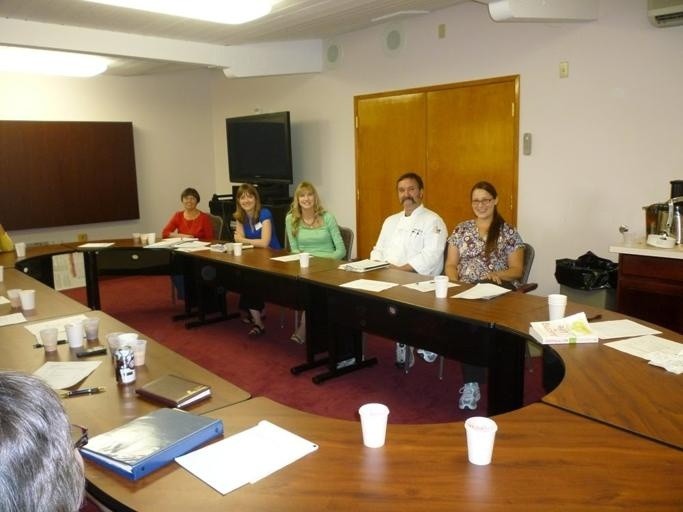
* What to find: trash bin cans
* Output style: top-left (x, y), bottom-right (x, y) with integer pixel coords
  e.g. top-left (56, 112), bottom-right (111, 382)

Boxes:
top-left (554, 251), bottom-right (618, 311)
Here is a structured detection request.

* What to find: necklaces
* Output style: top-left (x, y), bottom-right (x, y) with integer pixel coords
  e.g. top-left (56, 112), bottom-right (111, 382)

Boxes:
top-left (300, 214), bottom-right (317, 229)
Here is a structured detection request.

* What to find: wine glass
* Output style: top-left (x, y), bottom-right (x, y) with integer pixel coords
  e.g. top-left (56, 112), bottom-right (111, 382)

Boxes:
top-left (435, 275), bottom-right (449, 298)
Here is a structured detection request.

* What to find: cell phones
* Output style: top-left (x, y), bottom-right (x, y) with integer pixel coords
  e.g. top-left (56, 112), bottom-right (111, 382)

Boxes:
top-left (76, 346), bottom-right (106, 358)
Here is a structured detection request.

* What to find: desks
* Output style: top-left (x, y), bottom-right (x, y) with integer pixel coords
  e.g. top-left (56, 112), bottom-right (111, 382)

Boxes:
top-left (209, 200), bottom-right (293, 248)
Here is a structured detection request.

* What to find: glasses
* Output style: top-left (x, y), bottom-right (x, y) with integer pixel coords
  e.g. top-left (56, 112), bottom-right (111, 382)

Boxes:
top-left (472, 199), bottom-right (493, 205)
top-left (70, 423), bottom-right (87, 449)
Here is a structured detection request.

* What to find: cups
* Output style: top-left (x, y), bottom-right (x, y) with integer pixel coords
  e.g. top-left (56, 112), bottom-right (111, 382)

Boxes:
top-left (226, 242), bottom-right (243, 257)
top-left (15, 243), bottom-right (26, 257)
top-left (0, 266), bottom-right (4, 282)
top-left (7, 289), bottom-right (36, 310)
top-left (132, 232), bottom-right (156, 246)
top-left (39, 316), bottom-right (146, 367)
top-left (300, 253), bottom-right (309, 268)
top-left (464, 415), bottom-right (498, 466)
top-left (358, 402), bottom-right (390, 449)
top-left (548, 294), bottom-right (568, 321)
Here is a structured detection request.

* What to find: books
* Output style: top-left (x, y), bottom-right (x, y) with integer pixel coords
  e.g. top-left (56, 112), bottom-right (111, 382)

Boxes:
top-left (338, 259), bottom-right (390, 273)
top-left (135, 375), bottom-right (211, 410)
top-left (529, 312), bottom-right (598, 346)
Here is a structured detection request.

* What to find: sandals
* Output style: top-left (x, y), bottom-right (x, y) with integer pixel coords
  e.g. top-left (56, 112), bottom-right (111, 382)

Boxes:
top-left (240, 315), bottom-right (266, 324)
top-left (248, 325), bottom-right (265, 336)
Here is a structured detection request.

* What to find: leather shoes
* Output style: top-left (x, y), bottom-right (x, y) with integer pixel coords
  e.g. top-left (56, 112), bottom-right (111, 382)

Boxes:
top-left (291, 333), bottom-right (305, 345)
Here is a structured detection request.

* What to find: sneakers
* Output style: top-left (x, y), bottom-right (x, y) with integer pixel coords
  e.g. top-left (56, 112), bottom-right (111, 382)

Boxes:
top-left (396, 343), bottom-right (415, 368)
top-left (458, 382), bottom-right (481, 410)
top-left (417, 348), bottom-right (438, 363)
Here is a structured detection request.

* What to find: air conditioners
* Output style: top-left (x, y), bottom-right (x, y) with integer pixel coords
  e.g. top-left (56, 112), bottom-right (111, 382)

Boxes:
top-left (647, 1), bottom-right (683, 27)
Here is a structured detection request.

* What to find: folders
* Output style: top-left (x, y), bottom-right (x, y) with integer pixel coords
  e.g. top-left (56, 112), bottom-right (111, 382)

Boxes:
top-left (79, 408), bottom-right (223, 481)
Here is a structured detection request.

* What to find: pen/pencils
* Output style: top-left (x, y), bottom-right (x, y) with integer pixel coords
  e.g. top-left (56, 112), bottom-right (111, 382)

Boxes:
top-left (586, 314), bottom-right (601, 322)
top-left (76, 349), bottom-right (106, 357)
top-left (33, 340), bottom-right (66, 348)
top-left (59, 387), bottom-right (104, 396)
top-left (415, 281), bottom-right (434, 285)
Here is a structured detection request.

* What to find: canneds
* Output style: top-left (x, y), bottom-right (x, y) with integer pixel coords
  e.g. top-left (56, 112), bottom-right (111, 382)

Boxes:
top-left (113, 345), bottom-right (136, 386)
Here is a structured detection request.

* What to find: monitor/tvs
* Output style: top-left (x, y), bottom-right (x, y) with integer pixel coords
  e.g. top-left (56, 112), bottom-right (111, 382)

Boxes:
top-left (226, 111), bottom-right (293, 191)
top-left (0, 119), bottom-right (140, 232)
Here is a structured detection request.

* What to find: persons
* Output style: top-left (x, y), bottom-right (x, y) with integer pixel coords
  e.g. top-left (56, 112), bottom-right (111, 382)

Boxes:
top-left (370, 172), bottom-right (449, 369)
top-left (0, 374), bottom-right (87, 512)
top-left (232, 184), bottom-right (282, 337)
top-left (162, 188), bottom-right (228, 315)
top-left (444, 182), bottom-right (526, 411)
top-left (285, 182), bottom-right (346, 345)
